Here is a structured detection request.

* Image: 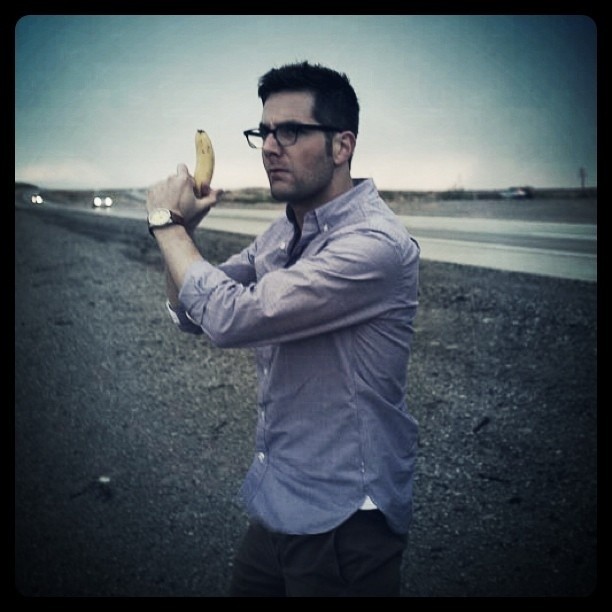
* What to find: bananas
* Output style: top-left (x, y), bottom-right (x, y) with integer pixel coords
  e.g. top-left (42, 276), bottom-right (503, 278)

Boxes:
top-left (196, 129), bottom-right (213, 194)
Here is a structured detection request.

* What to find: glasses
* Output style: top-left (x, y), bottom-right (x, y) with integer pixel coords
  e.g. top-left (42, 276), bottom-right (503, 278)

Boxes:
top-left (242, 124), bottom-right (343, 149)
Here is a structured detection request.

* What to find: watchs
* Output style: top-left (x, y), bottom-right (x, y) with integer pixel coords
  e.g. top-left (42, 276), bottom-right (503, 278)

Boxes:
top-left (146, 205), bottom-right (186, 232)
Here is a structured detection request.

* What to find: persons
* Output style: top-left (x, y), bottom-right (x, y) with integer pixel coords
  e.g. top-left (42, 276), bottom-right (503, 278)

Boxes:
top-left (142, 60), bottom-right (424, 597)
